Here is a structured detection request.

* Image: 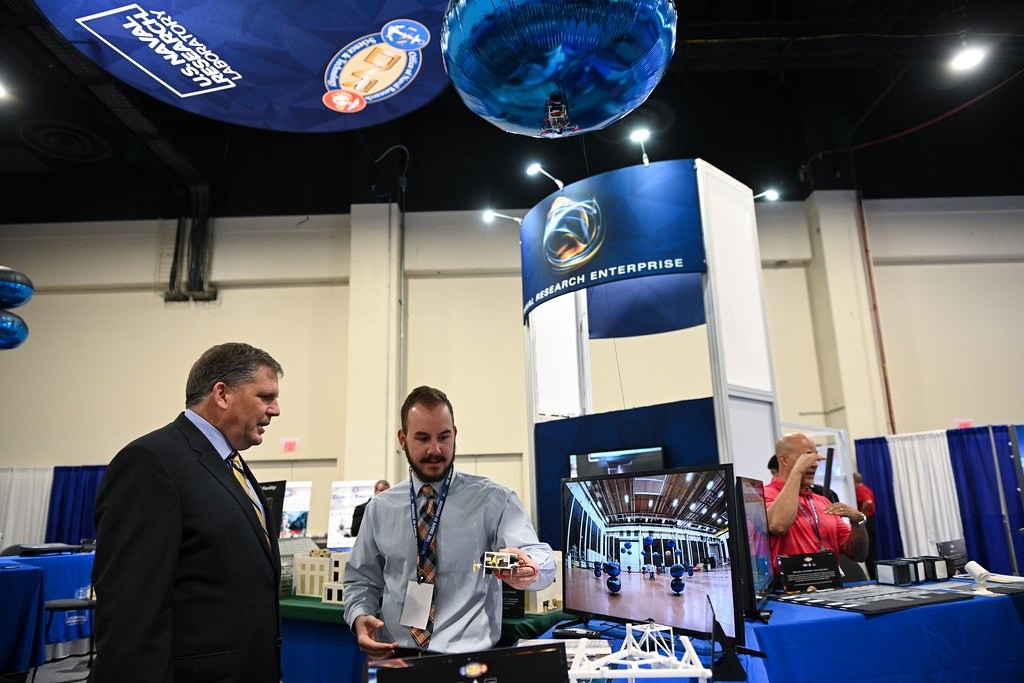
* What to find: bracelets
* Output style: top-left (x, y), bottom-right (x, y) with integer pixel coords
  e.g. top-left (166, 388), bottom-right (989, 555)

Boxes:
top-left (849, 511), bottom-right (867, 526)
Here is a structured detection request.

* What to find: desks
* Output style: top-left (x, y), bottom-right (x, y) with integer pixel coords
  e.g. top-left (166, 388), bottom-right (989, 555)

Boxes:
top-left (277, 574), bottom-right (1024, 683)
top-left (0, 549), bottom-right (97, 683)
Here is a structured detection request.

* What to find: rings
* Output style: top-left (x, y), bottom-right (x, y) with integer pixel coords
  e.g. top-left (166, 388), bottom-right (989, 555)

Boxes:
top-left (840, 508), bottom-right (843, 511)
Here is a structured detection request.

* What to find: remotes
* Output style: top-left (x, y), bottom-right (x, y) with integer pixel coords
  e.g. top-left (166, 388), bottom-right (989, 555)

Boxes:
top-left (553, 629), bottom-right (601, 640)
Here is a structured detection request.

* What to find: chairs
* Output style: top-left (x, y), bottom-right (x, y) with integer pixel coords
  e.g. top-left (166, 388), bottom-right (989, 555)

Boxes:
top-left (33, 580), bottom-right (96, 669)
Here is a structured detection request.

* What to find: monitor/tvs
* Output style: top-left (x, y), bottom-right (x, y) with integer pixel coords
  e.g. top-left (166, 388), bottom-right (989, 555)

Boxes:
top-left (561, 442), bottom-right (776, 647)
top-left (366, 642), bottom-right (570, 683)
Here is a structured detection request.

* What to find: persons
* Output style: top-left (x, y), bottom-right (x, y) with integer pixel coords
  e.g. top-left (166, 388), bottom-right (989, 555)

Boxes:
top-left (351, 480), bottom-right (390, 537)
top-left (764, 431), bottom-right (877, 590)
top-left (746, 513), bottom-right (768, 576)
top-left (87, 343), bottom-right (284, 683)
top-left (343, 386), bottom-right (558, 683)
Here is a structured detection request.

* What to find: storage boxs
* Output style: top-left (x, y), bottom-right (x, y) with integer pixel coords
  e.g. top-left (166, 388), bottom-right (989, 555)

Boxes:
top-left (873, 555), bottom-right (948, 586)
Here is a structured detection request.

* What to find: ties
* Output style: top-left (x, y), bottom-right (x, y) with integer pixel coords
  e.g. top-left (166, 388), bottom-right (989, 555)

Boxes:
top-left (411, 486), bottom-right (438, 648)
top-left (225, 451), bottom-right (271, 549)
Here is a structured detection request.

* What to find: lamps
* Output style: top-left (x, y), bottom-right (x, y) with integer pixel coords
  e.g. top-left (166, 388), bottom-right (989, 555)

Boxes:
top-left (528, 163), bottom-right (563, 191)
top-left (483, 208), bottom-right (522, 226)
top-left (754, 188), bottom-right (780, 202)
top-left (630, 129), bottom-right (650, 167)
